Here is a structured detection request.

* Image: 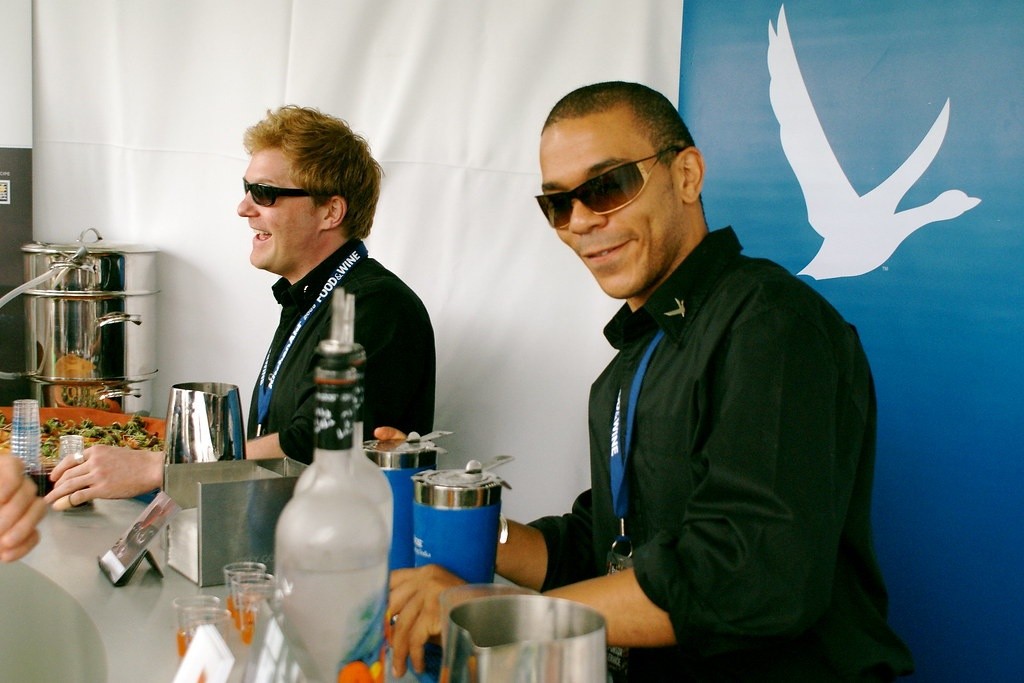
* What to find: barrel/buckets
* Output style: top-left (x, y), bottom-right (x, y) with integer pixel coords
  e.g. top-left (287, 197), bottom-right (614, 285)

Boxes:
top-left (20, 228), bottom-right (165, 417)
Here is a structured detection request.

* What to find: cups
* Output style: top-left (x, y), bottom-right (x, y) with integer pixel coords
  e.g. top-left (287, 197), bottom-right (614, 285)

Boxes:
top-left (172, 561), bottom-right (277, 666)
top-left (10, 399), bottom-right (43, 474)
top-left (407, 455), bottom-right (512, 683)
top-left (365, 429), bottom-right (457, 570)
top-left (59, 434), bottom-right (84, 460)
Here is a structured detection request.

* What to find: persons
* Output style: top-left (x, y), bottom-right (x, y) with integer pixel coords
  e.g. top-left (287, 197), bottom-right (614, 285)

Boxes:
top-left (0, 450), bottom-right (48, 564)
top-left (374, 81), bottom-right (913, 683)
top-left (43, 105), bottom-right (436, 511)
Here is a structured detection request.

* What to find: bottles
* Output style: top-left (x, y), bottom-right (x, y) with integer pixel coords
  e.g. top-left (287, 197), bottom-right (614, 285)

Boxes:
top-left (275, 286), bottom-right (394, 682)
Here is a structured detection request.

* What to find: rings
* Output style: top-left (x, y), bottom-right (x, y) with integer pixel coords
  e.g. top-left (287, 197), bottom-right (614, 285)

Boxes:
top-left (68, 493), bottom-right (78, 507)
top-left (74, 451), bottom-right (86, 465)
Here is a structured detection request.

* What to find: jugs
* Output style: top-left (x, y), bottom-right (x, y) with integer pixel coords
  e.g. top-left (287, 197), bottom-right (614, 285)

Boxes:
top-left (437, 592), bottom-right (607, 683)
top-left (162, 382), bottom-right (247, 496)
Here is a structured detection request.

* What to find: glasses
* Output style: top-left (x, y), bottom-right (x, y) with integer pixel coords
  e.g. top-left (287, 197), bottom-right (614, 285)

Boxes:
top-left (242, 178), bottom-right (312, 207)
top-left (536, 145), bottom-right (685, 230)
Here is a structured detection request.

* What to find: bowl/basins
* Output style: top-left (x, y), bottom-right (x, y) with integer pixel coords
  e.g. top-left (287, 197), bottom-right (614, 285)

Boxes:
top-left (0, 406), bottom-right (166, 496)
